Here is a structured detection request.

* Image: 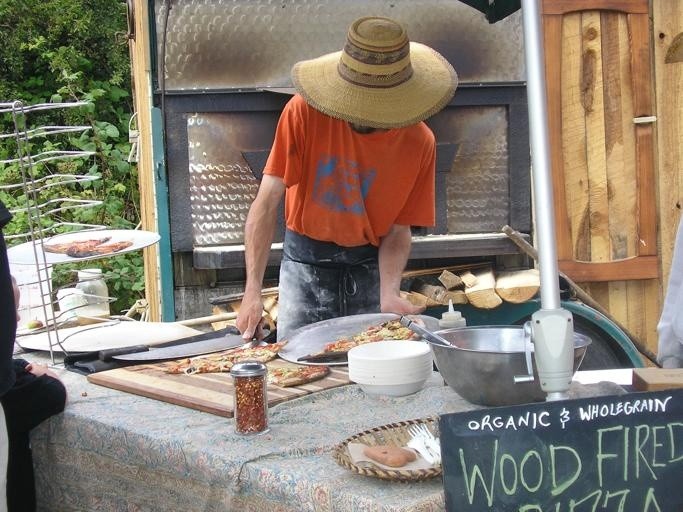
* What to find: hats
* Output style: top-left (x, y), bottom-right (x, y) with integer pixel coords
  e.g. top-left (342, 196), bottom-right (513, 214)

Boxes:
top-left (291, 17), bottom-right (458, 128)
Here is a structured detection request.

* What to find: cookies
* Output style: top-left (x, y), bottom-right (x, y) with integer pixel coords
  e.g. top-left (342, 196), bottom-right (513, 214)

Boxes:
top-left (365, 445), bottom-right (417, 468)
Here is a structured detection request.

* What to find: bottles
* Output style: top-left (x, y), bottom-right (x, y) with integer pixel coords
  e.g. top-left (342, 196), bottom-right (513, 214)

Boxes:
top-left (76, 269), bottom-right (110, 319)
top-left (229, 362), bottom-right (268, 437)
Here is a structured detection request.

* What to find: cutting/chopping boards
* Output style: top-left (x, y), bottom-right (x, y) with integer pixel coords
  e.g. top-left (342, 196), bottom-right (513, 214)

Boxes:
top-left (86, 341), bottom-right (357, 418)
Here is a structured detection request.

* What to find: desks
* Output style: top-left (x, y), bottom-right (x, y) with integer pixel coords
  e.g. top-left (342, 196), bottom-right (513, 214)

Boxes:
top-left (12, 341), bottom-right (628, 512)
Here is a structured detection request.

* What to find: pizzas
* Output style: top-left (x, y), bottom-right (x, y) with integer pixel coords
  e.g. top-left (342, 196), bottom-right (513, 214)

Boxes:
top-left (265, 365), bottom-right (330, 388)
top-left (42, 235), bottom-right (112, 254)
top-left (67, 241), bottom-right (133, 258)
top-left (164, 340), bottom-right (289, 373)
top-left (325, 318), bottom-right (424, 352)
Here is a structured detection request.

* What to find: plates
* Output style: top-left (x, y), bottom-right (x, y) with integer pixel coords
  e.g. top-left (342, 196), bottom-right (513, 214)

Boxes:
top-left (6, 228), bottom-right (160, 264)
top-left (346, 340), bottom-right (432, 397)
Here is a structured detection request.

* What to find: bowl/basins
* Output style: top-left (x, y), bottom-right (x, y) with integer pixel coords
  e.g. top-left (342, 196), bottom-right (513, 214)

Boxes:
top-left (421, 323), bottom-right (592, 407)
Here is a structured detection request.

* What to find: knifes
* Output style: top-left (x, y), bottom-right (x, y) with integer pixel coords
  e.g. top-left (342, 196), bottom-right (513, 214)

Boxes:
top-left (97, 328), bottom-right (253, 361)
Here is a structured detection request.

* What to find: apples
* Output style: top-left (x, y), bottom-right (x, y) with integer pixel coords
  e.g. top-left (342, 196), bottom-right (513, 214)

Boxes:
top-left (28, 316), bottom-right (43, 328)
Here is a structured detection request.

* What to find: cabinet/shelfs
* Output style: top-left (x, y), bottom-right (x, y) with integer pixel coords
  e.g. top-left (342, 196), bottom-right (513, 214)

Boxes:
top-left (1, 101), bottom-right (123, 366)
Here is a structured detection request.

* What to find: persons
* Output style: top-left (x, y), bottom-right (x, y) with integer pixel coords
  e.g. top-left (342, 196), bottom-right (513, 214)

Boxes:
top-left (234, 93), bottom-right (459, 341)
top-left (0, 273), bottom-right (66, 511)
top-left (654, 216), bottom-right (682, 370)
